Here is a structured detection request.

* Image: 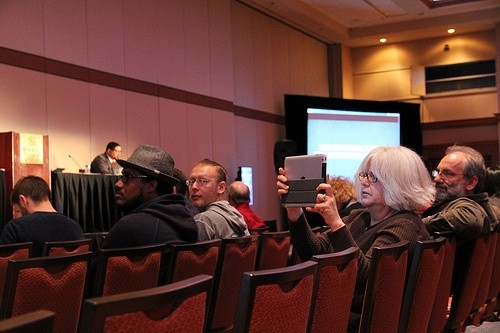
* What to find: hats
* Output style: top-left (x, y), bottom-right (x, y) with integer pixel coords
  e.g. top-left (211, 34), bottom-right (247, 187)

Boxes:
top-left (115, 143), bottom-right (181, 185)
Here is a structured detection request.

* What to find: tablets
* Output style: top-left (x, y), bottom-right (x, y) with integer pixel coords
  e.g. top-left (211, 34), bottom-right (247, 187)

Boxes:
top-left (282, 156), bottom-right (328, 207)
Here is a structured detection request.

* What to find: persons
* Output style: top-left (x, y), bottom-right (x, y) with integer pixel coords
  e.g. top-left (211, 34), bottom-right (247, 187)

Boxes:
top-left (419, 145), bottom-right (499, 236)
top-left (89, 141), bottom-right (122, 174)
top-left (276, 144), bottom-right (438, 333)
top-left (0, 175), bottom-right (84, 242)
top-left (483, 164), bottom-right (500, 220)
top-left (226, 181), bottom-right (268, 235)
top-left (101, 144), bottom-right (251, 250)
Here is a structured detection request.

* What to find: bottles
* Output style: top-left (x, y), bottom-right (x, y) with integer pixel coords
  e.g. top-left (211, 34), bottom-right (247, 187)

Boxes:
top-left (84, 165), bottom-right (90, 174)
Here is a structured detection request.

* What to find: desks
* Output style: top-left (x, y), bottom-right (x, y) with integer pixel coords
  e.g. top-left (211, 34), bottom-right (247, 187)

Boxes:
top-left (51, 171), bottom-right (127, 231)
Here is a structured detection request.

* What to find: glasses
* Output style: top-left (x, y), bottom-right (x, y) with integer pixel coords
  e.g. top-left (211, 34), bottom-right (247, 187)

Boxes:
top-left (185, 178), bottom-right (222, 187)
top-left (358, 172), bottom-right (378, 183)
top-left (431, 168), bottom-right (466, 180)
top-left (121, 171), bottom-right (150, 183)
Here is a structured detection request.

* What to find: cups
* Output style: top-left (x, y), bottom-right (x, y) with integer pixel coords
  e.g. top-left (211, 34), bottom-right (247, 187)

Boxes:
top-left (79, 168), bottom-right (85, 174)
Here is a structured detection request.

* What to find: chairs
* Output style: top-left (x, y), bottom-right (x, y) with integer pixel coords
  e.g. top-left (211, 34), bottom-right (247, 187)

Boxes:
top-left (0, 220), bottom-right (500, 333)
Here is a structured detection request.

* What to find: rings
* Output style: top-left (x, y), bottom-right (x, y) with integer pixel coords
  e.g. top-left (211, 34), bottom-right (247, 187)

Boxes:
top-left (322, 194), bottom-right (327, 201)
